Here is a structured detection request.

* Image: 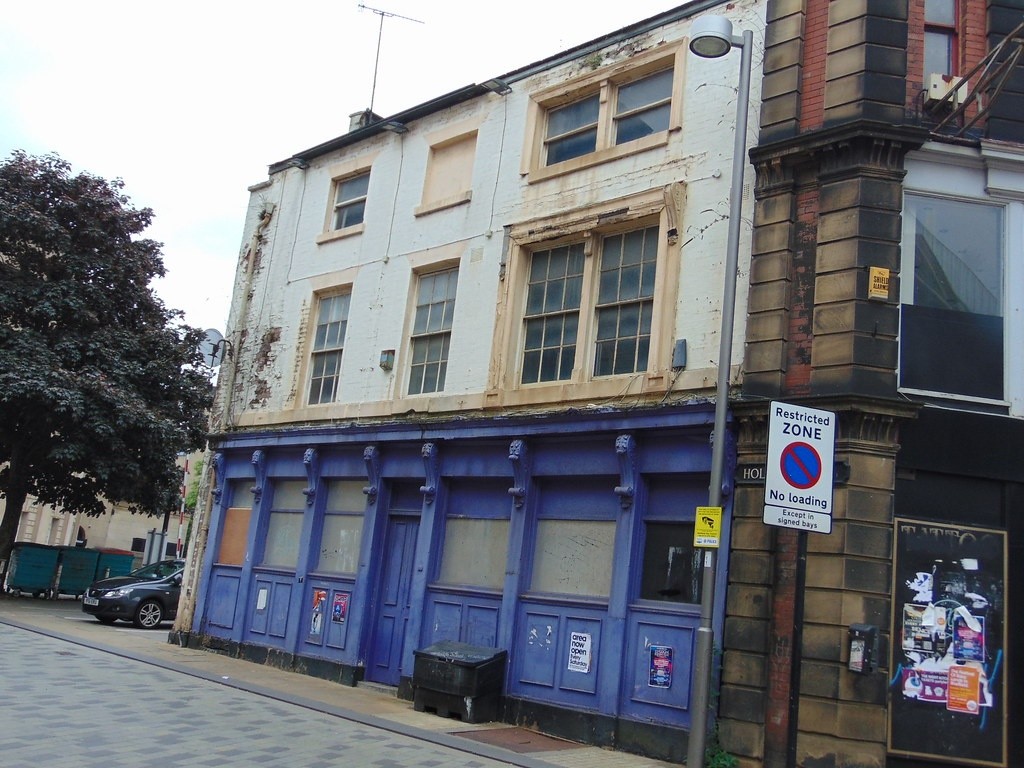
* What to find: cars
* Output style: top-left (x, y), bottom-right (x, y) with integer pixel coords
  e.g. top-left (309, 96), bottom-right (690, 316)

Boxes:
top-left (81, 559), bottom-right (185, 630)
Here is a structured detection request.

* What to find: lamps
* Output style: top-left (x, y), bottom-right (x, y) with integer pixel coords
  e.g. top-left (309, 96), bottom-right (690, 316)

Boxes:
top-left (481, 78), bottom-right (512, 97)
top-left (382, 121), bottom-right (410, 134)
top-left (288, 158), bottom-right (310, 169)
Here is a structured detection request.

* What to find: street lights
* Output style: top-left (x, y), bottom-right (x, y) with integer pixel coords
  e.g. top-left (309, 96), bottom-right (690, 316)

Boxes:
top-left (686, 13), bottom-right (756, 768)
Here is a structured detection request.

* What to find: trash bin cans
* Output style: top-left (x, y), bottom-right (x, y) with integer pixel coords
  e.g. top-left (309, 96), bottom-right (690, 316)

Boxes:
top-left (413, 640), bottom-right (506, 725)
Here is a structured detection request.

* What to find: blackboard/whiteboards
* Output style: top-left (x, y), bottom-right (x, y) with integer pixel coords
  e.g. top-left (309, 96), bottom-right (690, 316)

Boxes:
top-left (885, 516), bottom-right (1009, 767)
